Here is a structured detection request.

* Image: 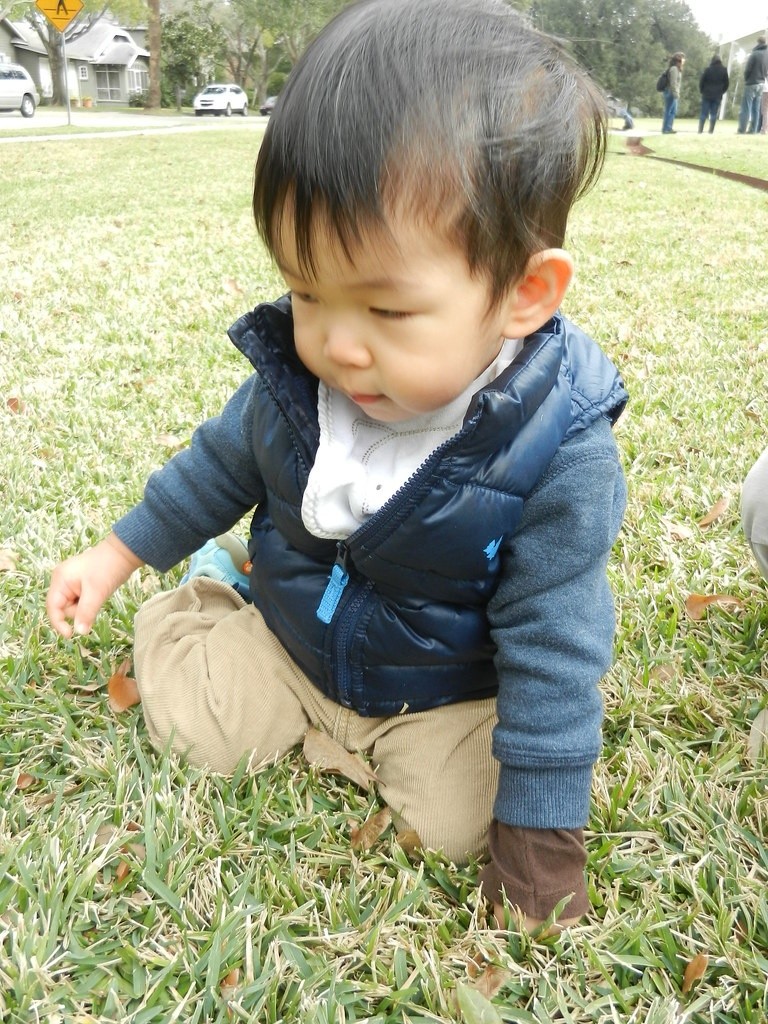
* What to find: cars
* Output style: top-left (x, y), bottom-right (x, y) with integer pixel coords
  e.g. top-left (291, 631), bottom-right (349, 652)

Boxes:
top-left (258, 96), bottom-right (277, 115)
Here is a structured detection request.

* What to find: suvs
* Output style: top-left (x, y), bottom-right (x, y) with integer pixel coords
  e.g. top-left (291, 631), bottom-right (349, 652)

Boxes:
top-left (194, 84), bottom-right (248, 117)
top-left (0, 62), bottom-right (40, 118)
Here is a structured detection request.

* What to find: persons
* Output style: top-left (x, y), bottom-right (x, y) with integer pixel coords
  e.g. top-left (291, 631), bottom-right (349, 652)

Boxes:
top-left (661, 51), bottom-right (685, 134)
top-left (695, 52), bottom-right (729, 134)
top-left (43, 0), bottom-right (635, 944)
top-left (734, 34), bottom-right (768, 132)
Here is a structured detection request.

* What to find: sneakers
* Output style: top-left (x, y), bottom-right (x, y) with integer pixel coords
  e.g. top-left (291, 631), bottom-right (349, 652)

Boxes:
top-left (179, 535), bottom-right (254, 598)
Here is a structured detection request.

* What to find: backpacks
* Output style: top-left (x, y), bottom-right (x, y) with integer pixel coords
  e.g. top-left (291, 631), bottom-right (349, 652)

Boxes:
top-left (656, 65), bottom-right (670, 92)
